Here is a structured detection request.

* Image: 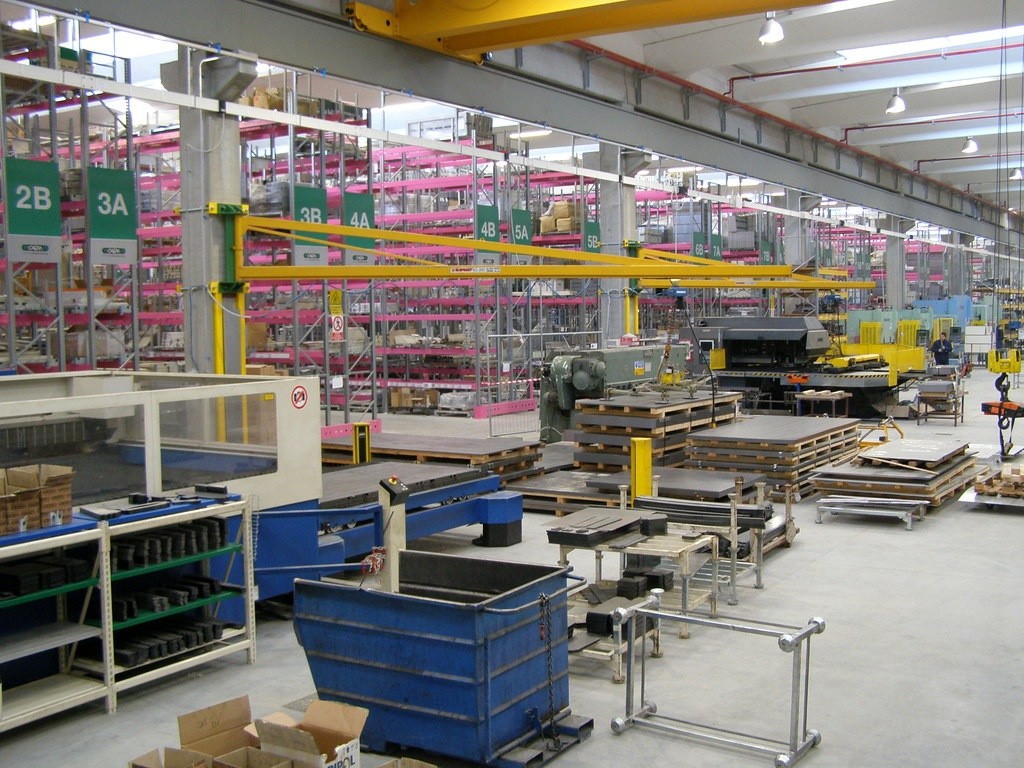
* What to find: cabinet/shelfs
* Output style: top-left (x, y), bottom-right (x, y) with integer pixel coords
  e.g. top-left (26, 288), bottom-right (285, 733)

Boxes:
top-left (556, 471), bottom-right (794, 684)
top-left (0, 496), bottom-right (257, 734)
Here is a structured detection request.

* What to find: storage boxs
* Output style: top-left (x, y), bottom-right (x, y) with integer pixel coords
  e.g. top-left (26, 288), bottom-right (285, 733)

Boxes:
top-left (0, 464), bottom-right (42, 536)
top-left (177, 695), bottom-right (261, 759)
top-left (872, 397), bottom-right (935, 419)
top-left (538, 216), bottom-right (558, 233)
top-left (643, 227), bottom-right (669, 244)
top-left (128, 746), bottom-right (213, 768)
top-left (37, 464), bottom-right (78, 528)
top-left (244, 698), bottom-right (369, 768)
top-left (239, 86), bottom-right (319, 115)
top-left (556, 217), bottom-right (575, 231)
top-left (553, 201), bottom-right (573, 218)
top-left (213, 745), bottom-right (293, 768)
top-left (377, 757), bottom-right (438, 768)
top-left (46, 328), bottom-right (127, 360)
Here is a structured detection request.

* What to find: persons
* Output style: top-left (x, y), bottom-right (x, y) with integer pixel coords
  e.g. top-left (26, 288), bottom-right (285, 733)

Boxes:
top-left (996, 324), bottom-right (1007, 349)
top-left (930, 332), bottom-right (952, 366)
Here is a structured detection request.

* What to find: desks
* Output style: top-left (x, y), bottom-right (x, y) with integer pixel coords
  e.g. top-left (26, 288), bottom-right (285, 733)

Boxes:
top-left (916, 392), bottom-right (969, 426)
top-left (794, 390), bottom-right (853, 418)
